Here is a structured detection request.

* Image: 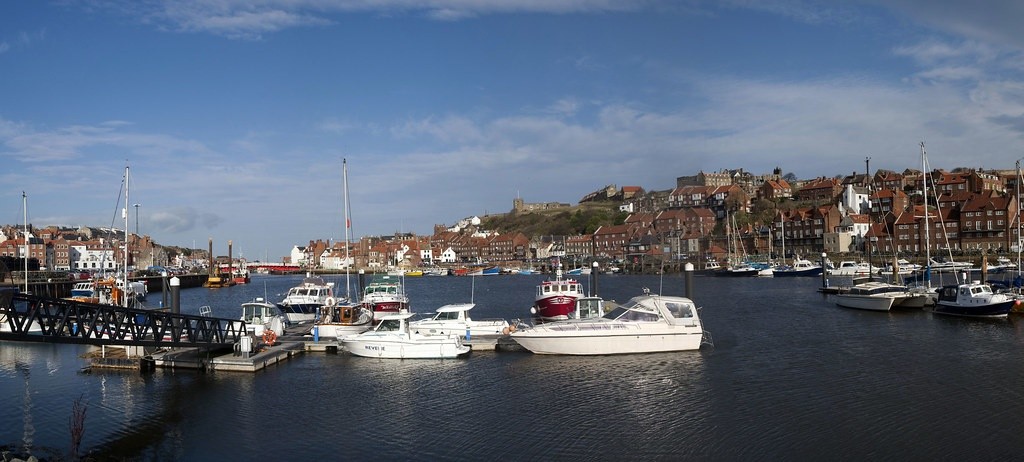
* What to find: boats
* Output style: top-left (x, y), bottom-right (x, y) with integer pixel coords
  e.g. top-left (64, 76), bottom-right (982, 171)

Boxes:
top-left (307, 294), bottom-right (375, 340)
top-left (68, 161), bottom-right (149, 305)
top-left (337, 329), bottom-right (473, 360)
top-left (406, 300), bottom-right (509, 352)
top-left (385, 265), bottom-right (592, 276)
top-left (225, 293), bottom-right (285, 340)
top-left (509, 293), bottom-right (704, 357)
top-left (531, 257), bottom-right (587, 321)
top-left (233, 267), bottom-right (249, 284)
top-left (361, 278), bottom-right (412, 321)
top-left (81, 322), bottom-right (194, 342)
top-left (276, 272), bottom-right (336, 325)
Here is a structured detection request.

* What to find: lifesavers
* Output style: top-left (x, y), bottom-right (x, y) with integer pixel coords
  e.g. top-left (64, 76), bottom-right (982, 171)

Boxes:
top-left (323, 296), bottom-right (336, 307)
top-left (262, 330), bottom-right (277, 346)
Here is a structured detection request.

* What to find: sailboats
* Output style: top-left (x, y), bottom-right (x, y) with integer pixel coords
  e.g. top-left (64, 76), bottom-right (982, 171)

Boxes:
top-left (714, 141), bottom-right (1024, 321)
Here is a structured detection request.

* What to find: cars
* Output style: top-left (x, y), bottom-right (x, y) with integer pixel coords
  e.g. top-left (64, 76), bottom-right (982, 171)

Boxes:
top-left (54, 264), bottom-right (187, 280)
